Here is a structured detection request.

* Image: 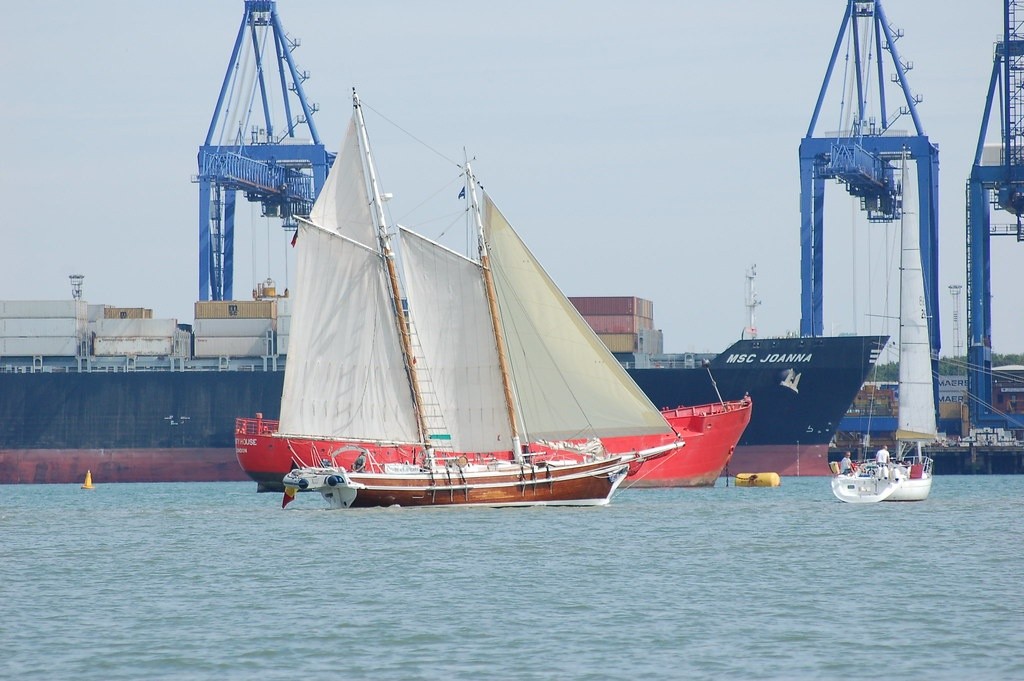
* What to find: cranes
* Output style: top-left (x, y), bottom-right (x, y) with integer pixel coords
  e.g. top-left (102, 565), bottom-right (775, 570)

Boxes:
top-left (799, 0), bottom-right (959, 434)
top-left (191, 0), bottom-right (339, 302)
top-left (969, 0), bottom-right (1023, 436)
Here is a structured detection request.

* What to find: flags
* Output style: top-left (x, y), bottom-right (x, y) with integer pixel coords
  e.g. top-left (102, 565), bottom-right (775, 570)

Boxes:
top-left (282, 461), bottom-right (298, 509)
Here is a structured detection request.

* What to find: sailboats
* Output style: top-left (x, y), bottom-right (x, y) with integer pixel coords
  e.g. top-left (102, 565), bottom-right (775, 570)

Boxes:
top-left (829, 145), bottom-right (936, 503)
top-left (279, 87), bottom-right (687, 511)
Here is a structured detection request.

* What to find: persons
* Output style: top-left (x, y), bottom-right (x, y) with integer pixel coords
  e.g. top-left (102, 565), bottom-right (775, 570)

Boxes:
top-left (355, 452), bottom-right (366, 473)
top-left (841, 451), bottom-right (852, 475)
top-left (876, 446), bottom-right (890, 467)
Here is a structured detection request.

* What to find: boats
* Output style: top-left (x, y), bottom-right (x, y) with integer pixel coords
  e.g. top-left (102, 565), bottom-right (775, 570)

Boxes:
top-left (233, 395), bottom-right (753, 494)
